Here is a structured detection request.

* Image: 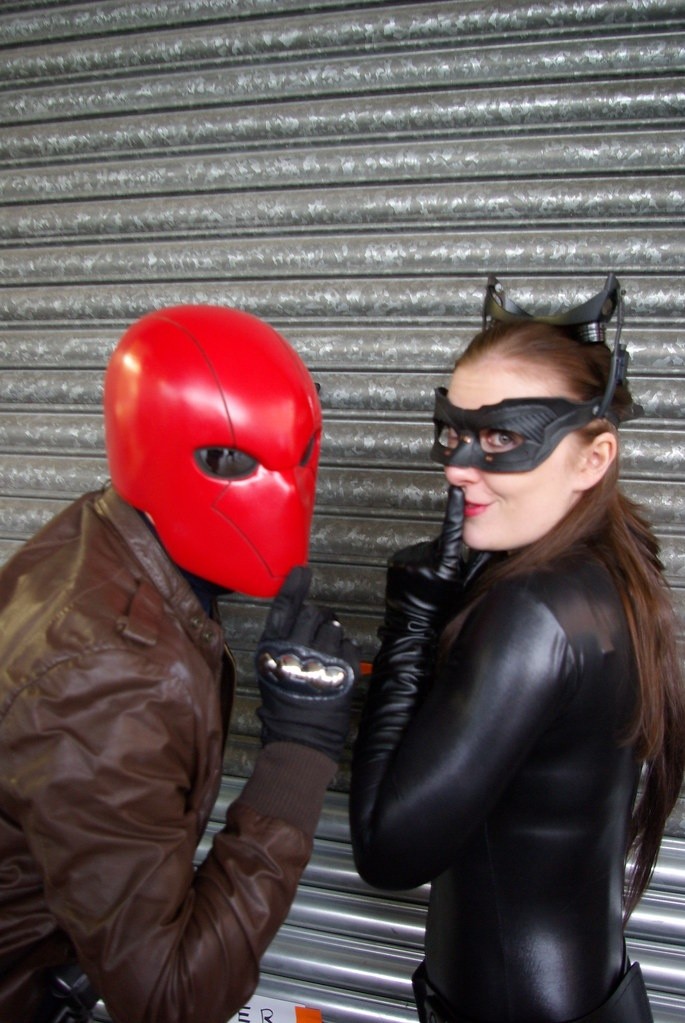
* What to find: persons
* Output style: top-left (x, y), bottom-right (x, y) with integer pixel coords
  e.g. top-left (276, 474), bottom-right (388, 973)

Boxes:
top-left (350, 271), bottom-right (684, 1023)
top-left (0, 306), bottom-right (360, 1023)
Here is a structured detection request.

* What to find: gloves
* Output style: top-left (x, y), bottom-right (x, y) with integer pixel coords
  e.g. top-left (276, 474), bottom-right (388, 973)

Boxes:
top-left (352, 486), bottom-right (491, 753)
top-left (255, 565), bottom-right (361, 763)
